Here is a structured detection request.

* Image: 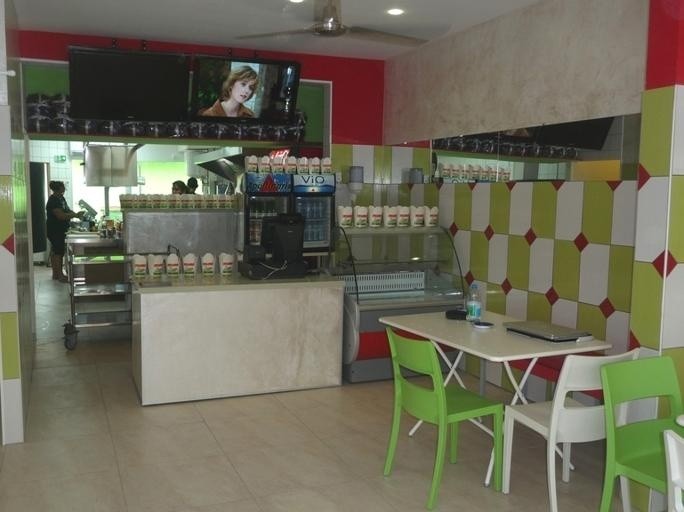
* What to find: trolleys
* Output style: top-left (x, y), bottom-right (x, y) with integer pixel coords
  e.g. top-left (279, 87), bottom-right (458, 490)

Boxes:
top-left (62, 251), bottom-right (133, 351)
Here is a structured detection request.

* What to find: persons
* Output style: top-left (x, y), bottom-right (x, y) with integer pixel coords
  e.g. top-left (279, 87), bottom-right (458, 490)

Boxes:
top-left (46, 180), bottom-right (85, 281)
top-left (172, 180), bottom-right (189, 194)
top-left (188, 175), bottom-right (199, 194)
top-left (196, 65), bottom-right (260, 118)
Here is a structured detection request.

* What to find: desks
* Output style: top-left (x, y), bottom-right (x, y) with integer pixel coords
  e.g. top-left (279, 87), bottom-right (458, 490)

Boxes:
top-left (377, 310), bottom-right (615, 488)
top-left (63, 231), bottom-right (125, 279)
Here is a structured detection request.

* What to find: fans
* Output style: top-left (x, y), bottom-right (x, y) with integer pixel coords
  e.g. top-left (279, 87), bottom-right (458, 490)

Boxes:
top-left (232, 0), bottom-right (431, 51)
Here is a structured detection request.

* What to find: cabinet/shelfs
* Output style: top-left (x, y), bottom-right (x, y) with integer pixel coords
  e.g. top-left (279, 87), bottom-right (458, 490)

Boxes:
top-left (64, 253), bottom-right (130, 330)
top-left (129, 274), bottom-right (349, 410)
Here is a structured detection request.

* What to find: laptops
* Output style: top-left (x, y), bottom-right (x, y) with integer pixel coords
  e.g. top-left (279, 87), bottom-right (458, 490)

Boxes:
top-left (503, 320), bottom-right (588, 340)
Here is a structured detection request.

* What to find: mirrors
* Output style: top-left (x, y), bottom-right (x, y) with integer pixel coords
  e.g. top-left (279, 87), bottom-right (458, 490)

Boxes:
top-left (429, 130), bottom-right (499, 185)
top-left (351, 140), bottom-right (431, 185)
top-left (497, 112), bottom-right (643, 183)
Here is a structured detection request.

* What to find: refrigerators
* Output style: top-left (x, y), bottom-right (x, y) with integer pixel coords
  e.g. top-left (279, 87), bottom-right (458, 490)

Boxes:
top-left (234, 172), bottom-right (336, 256)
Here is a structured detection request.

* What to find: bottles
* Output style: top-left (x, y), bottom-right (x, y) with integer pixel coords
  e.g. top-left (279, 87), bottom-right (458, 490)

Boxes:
top-left (101, 216), bottom-right (108, 229)
top-left (465, 284), bottom-right (480, 322)
top-left (303, 221), bottom-right (324, 241)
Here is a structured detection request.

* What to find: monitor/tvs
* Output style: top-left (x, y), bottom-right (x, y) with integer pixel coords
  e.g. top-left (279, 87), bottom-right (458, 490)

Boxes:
top-left (263, 216), bottom-right (302, 260)
top-left (69, 49), bottom-right (191, 122)
top-left (191, 56), bottom-right (301, 125)
top-left (536, 117), bottom-right (616, 150)
top-left (478, 126), bottom-right (541, 144)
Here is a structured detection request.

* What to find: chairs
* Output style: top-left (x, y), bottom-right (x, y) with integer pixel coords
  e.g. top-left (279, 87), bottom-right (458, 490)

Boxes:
top-left (500, 348), bottom-right (643, 512)
top-left (596, 354), bottom-right (684, 512)
top-left (380, 325), bottom-right (502, 511)
